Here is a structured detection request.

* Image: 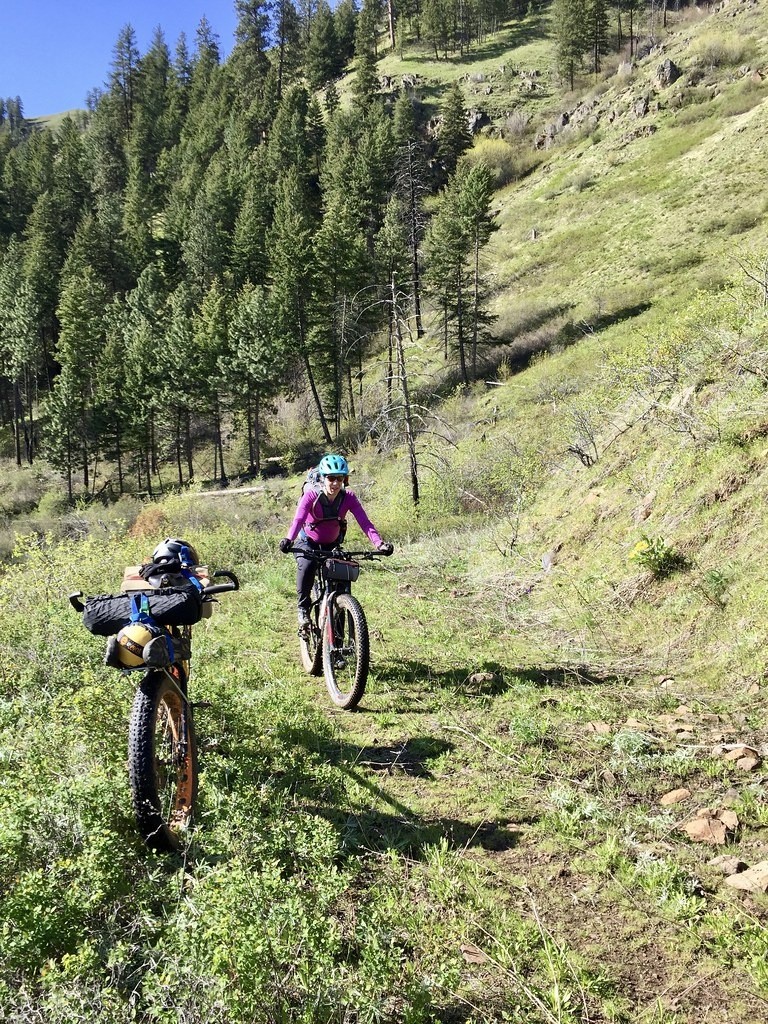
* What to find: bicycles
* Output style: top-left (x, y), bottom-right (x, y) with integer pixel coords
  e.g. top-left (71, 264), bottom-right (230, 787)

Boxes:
top-left (67, 570), bottom-right (241, 858)
top-left (284, 546), bottom-right (388, 710)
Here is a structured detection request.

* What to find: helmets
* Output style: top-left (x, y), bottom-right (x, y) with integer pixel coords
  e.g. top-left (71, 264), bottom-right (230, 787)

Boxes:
top-left (153, 538), bottom-right (199, 565)
top-left (319, 455), bottom-right (349, 476)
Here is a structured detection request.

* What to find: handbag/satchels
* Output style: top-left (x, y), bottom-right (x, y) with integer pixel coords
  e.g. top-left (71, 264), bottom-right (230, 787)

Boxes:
top-left (117, 592), bottom-right (172, 670)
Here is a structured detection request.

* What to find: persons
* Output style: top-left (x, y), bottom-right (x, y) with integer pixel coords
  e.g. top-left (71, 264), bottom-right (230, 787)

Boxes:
top-left (279, 455), bottom-right (394, 669)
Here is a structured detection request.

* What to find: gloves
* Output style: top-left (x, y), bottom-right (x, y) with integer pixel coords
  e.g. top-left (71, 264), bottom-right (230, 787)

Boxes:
top-left (377, 542), bottom-right (394, 556)
top-left (139, 558), bottom-right (178, 581)
top-left (279, 538), bottom-right (294, 554)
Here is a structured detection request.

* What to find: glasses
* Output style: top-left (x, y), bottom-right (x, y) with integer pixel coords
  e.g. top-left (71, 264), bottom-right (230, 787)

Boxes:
top-left (326, 476), bottom-right (344, 482)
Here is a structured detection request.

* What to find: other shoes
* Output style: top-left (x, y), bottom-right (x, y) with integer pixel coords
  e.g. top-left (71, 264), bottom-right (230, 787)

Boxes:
top-left (335, 657), bottom-right (346, 667)
top-left (105, 636), bottom-right (132, 675)
top-left (143, 635), bottom-right (191, 666)
top-left (298, 607), bottom-right (313, 625)
